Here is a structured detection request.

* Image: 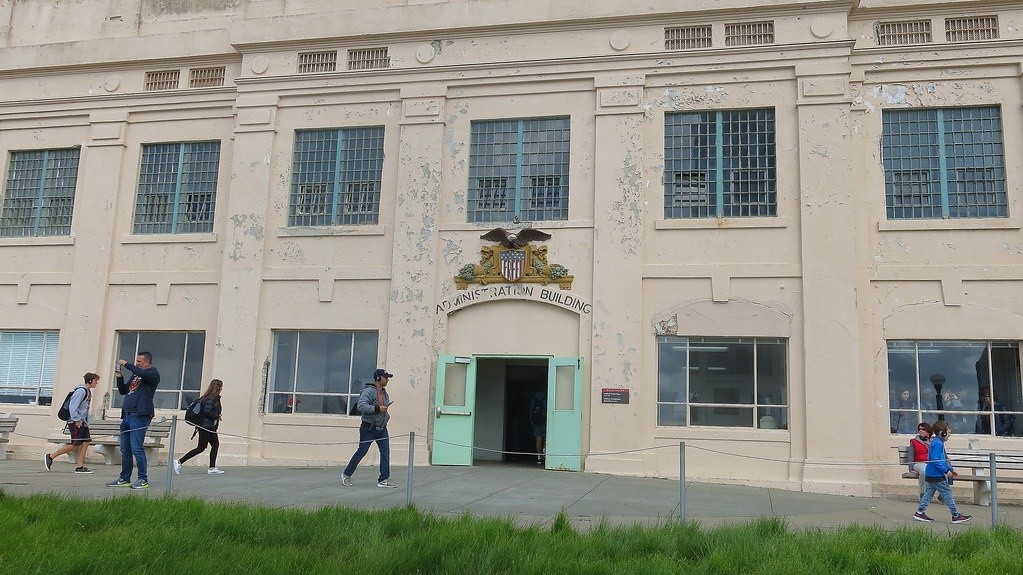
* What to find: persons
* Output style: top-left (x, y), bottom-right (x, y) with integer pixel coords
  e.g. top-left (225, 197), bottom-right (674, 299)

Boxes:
top-left (908, 422), bottom-right (947, 506)
top-left (104, 351), bottom-right (161, 489)
top-left (173, 379), bottom-right (226, 475)
top-left (912, 421), bottom-right (972, 524)
top-left (44, 372), bottom-right (102, 473)
top-left (339, 369), bottom-right (397, 489)
top-left (891, 385), bottom-right (1016, 437)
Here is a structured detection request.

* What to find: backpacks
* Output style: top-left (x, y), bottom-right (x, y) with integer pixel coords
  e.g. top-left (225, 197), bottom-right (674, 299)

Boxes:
top-left (185, 397), bottom-right (204, 426)
top-left (58, 387), bottom-right (88, 421)
top-left (532, 396), bottom-right (546, 425)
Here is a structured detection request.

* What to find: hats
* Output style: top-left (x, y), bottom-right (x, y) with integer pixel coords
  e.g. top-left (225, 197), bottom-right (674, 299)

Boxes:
top-left (373, 369), bottom-right (393, 379)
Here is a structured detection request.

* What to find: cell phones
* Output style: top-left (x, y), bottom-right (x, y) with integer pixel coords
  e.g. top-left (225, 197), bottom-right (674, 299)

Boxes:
top-left (386, 401), bottom-right (394, 408)
top-left (115, 361), bottom-right (122, 373)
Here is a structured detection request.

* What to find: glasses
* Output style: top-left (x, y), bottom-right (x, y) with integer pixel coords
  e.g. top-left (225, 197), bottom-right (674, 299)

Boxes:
top-left (918, 429), bottom-right (925, 430)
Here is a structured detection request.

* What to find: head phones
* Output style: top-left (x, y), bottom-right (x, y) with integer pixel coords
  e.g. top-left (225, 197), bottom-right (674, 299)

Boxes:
top-left (919, 434), bottom-right (931, 441)
top-left (940, 421), bottom-right (950, 438)
top-left (376, 369), bottom-right (382, 381)
top-left (87, 373), bottom-right (93, 384)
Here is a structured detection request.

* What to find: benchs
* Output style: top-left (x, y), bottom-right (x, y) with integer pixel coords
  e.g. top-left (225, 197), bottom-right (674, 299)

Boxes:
top-left (47, 415), bottom-right (172, 466)
top-left (0, 412), bottom-right (18, 460)
top-left (898, 441), bottom-right (1023, 506)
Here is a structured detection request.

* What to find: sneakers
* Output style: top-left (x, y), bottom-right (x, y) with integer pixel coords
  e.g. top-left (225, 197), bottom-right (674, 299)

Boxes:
top-left (951, 513), bottom-right (972, 523)
top-left (340, 473), bottom-right (353, 487)
top-left (45, 453), bottom-right (53, 471)
top-left (74, 465), bottom-right (93, 474)
top-left (173, 460), bottom-right (183, 475)
top-left (130, 478), bottom-right (149, 489)
top-left (913, 511), bottom-right (934, 522)
top-left (207, 467), bottom-right (225, 475)
top-left (536, 459), bottom-right (543, 465)
top-left (376, 478), bottom-right (398, 488)
top-left (106, 476), bottom-right (132, 487)
